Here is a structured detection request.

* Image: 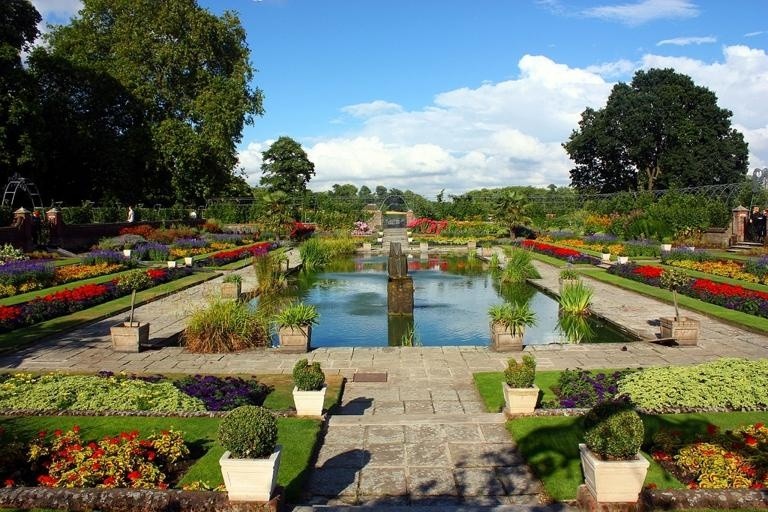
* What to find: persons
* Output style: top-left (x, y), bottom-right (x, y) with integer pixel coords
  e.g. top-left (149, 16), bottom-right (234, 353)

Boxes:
top-left (126, 206), bottom-right (135, 223)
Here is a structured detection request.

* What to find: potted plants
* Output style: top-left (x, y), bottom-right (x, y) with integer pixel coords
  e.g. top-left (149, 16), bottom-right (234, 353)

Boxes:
top-left (274, 300), bottom-right (321, 354)
top-left (558, 268), bottom-right (582, 292)
top-left (292, 358), bottom-right (328, 416)
top-left (216, 405), bottom-right (283, 501)
top-left (658, 265), bottom-right (701, 349)
top-left (486, 300), bottom-right (539, 353)
top-left (501, 354), bottom-right (540, 416)
top-left (110, 268), bottom-right (153, 353)
top-left (219, 273), bottom-right (243, 300)
top-left (576, 400), bottom-right (651, 504)
